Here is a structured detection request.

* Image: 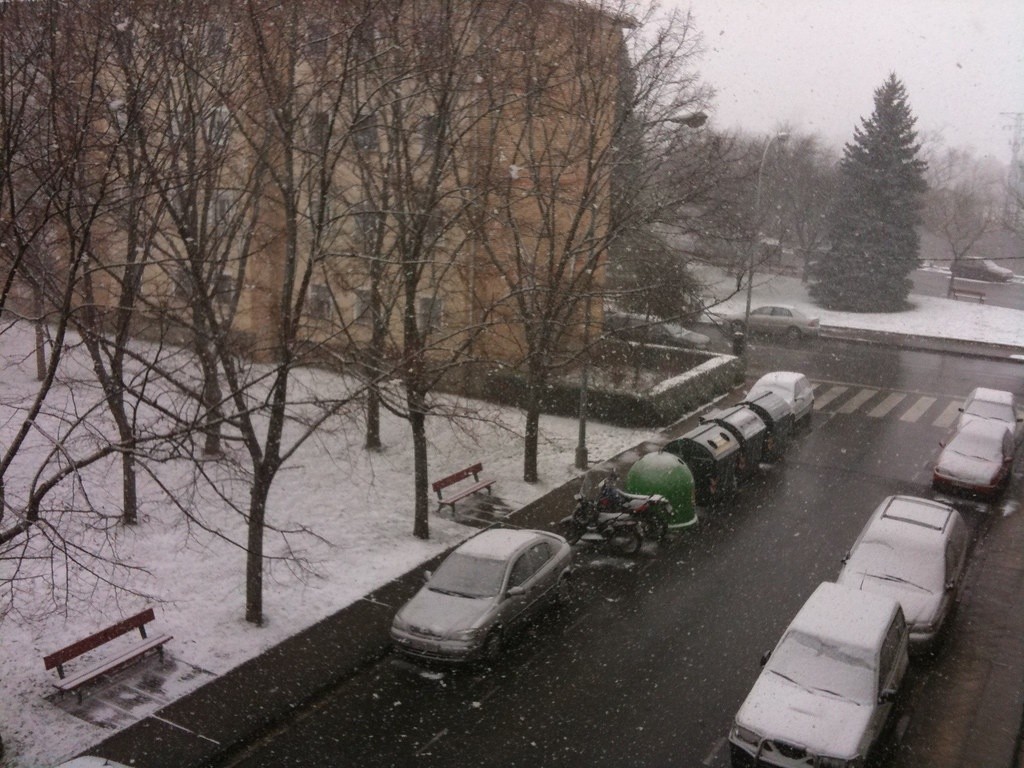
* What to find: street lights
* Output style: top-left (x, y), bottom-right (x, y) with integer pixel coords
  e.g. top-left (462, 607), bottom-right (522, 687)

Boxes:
top-left (574, 110), bottom-right (709, 471)
top-left (749, 132), bottom-right (789, 249)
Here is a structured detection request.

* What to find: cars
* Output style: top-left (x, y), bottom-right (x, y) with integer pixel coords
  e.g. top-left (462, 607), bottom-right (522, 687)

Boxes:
top-left (933, 418), bottom-right (1016, 500)
top-left (388, 528), bottom-right (574, 671)
top-left (957, 388), bottom-right (1023, 443)
top-left (742, 372), bottom-right (816, 435)
top-left (725, 304), bottom-right (820, 339)
top-left (836, 493), bottom-right (971, 647)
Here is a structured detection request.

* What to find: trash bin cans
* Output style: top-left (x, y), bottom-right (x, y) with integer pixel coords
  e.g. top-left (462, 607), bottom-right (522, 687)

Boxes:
top-left (699, 404), bottom-right (767, 481)
top-left (665, 422), bottom-right (742, 505)
top-left (627, 449), bottom-right (699, 531)
top-left (734, 389), bottom-right (793, 464)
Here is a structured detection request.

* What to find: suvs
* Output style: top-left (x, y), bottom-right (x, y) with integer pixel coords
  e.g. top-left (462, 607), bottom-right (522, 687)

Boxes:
top-left (949, 256), bottom-right (1014, 283)
top-left (728, 580), bottom-right (914, 768)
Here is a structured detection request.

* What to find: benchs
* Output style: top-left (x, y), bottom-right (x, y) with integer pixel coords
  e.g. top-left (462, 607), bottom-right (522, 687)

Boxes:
top-left (951, 287), bottom-right (986, 304)
top-left (432, 463), bottom-right (496, 517)
top-left (44, 608), bottom-right (174, 707)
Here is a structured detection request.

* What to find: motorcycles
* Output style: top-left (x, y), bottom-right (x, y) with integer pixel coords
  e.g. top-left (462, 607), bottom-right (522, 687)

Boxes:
top-left (593, 466), bottom-right (676, 545)
top-left (553, 493), bottom-right (646, 556)
